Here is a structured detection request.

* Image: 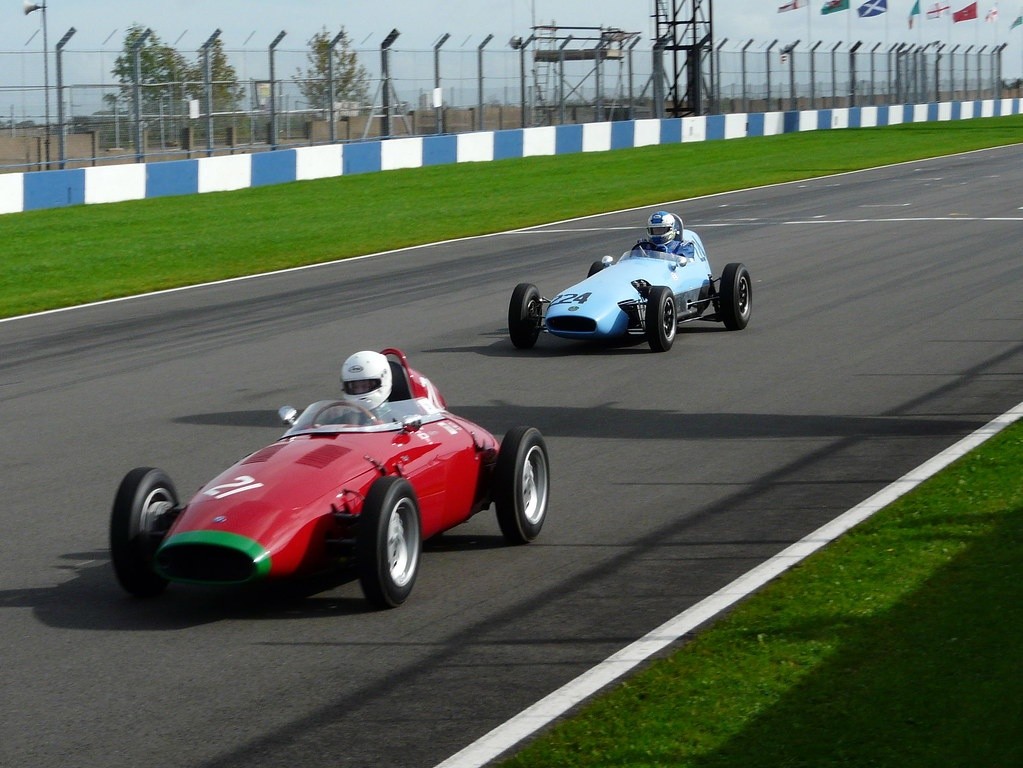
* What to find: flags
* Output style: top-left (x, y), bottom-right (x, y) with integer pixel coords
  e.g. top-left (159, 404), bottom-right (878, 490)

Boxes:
top-left (1009, 16), bottom-right (1023, 30)
top-left (985, 6), bottom-right (998, 23)
top-left (779, 0), bottom-right (809, 13)
top-left (954, 2), bottom-right (976, 22)
top-left (925, 1), bottom-right (951, 20)
top-left (821, 0), bottom-right (850, 14)
top-left (857, 0), bottom-right (887, 16)
top-left (909, 0), bottom-right (920, 28)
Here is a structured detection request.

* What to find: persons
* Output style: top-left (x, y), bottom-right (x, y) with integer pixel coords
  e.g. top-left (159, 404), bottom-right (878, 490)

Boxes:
top-left (303, 351), bottom-right (392, 427)
top-left (628, 212), bottom-right (694, 260)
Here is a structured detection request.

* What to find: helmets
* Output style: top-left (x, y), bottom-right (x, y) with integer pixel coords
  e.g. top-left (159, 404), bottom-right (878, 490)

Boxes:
top-left (646, 210), bottom-right (676, 246)
top-left (340, 350), bottom-right (393, 414)
